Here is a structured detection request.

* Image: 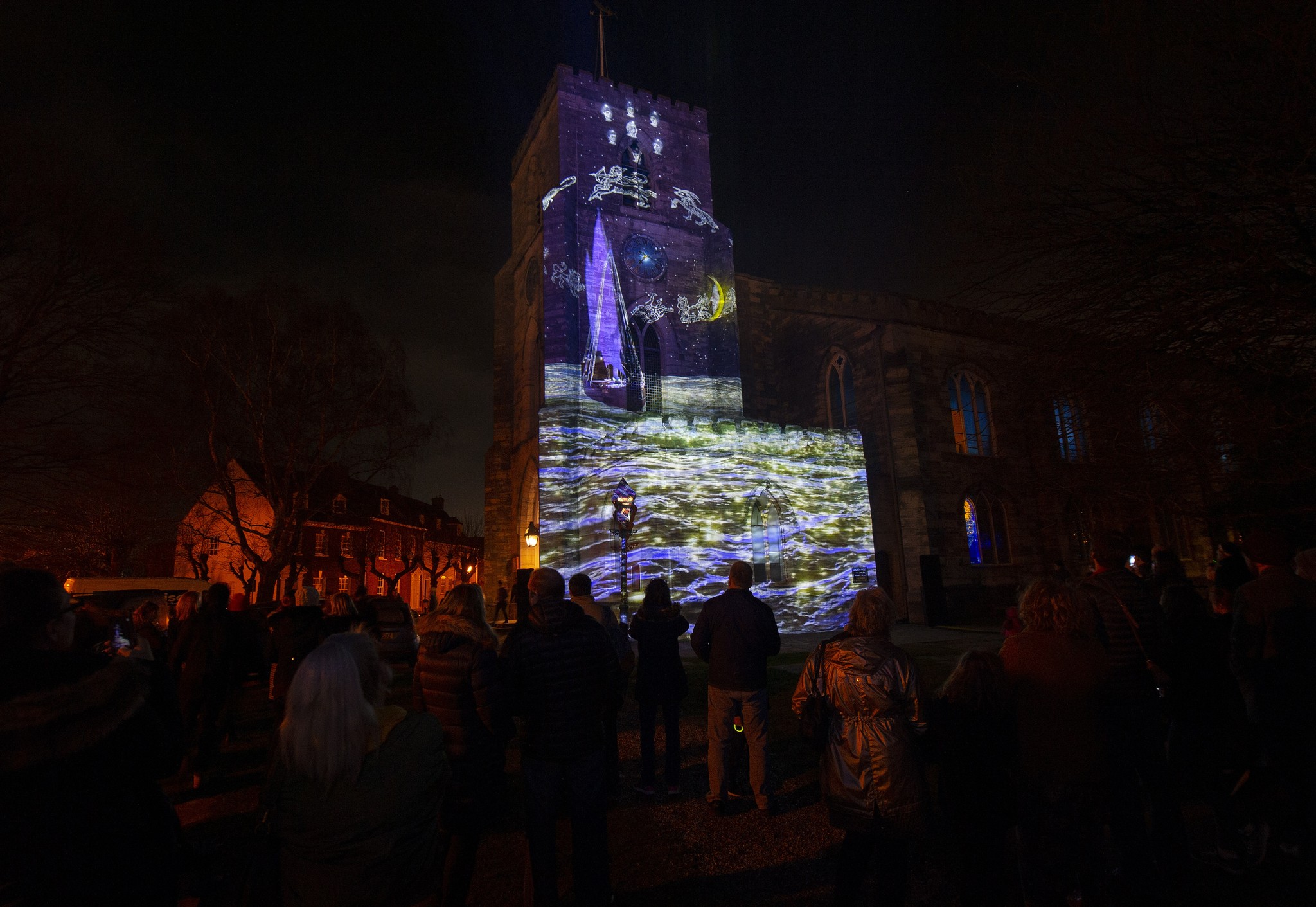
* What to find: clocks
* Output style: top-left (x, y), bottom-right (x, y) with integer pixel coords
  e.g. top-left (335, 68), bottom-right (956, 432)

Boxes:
top-left (621, 233), bottom-right (668, 284)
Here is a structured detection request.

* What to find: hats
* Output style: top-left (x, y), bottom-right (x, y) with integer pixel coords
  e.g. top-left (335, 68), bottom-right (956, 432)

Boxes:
top-left (1242, 527), bottom-right (1292, 566)
top-left (1295, 548), bottom-right (1316, 581)
top-left (1219, 540), bottom-right (1235, 556)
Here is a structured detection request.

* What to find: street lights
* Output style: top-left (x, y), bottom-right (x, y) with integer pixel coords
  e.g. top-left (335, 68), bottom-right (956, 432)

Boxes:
top-left (608, 476), bottom-right (640, 637)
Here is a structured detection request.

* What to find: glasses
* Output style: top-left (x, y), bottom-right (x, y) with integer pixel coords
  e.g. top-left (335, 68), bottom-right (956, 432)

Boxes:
top-left (60, 596), bottom-right (85, 613)
top-left (650, 578), bottom-right (668, 584)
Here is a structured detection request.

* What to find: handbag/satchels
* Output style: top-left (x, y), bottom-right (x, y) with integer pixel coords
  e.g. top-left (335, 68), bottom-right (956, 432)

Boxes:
top-left (801, 693), bottom-right (828, 753)
top-left (1147, 660), bottom-right (1176, 698)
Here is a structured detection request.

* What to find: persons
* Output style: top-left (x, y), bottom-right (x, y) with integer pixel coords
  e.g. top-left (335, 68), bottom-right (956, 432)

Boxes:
top-left (0, 565), bottom-right (178, 907)
top-left (66, 569), bottom-right (686, 801)
top-left (271, 629), bottom-right (448, 907)
top-left (792, 586), bottom-right (930, 907)
top-left (495, 568), bottom-right (625, 907)
top-left (414, 583), bottom-right (517, 907)
top-left (691, 561), bottom-right (781, 815)
top-left (922, 527), bottom-right (1316, 907)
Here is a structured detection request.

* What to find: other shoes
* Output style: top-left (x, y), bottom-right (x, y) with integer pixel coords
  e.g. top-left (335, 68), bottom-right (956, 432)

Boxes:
top-left (503, 620), bottom-right (509, 624)
top-left (491, 619), bottom-right (497, 623)
top-left (756, 801), bottom-right (773, 819)
top-left (706, 794), bottom-right (724, 815)
